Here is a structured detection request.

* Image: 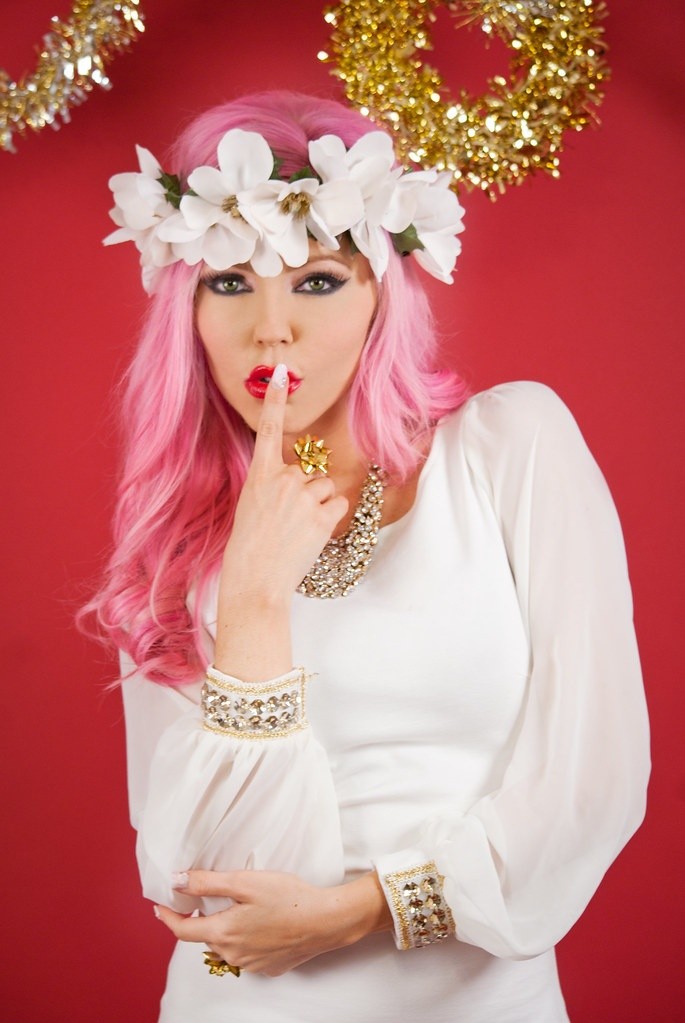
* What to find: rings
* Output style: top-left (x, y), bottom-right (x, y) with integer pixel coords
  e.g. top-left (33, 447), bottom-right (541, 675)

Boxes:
top-left (202, 952), bottom-right (245, 977)
top-left (293, 433), bottom-right (332, 475)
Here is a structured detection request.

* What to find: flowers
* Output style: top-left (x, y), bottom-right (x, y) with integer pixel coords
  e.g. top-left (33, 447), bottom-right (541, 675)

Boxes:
top-left (100, 126), bottom-right (464, 297)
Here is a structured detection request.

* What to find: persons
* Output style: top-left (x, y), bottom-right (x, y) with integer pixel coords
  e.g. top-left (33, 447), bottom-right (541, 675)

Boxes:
top-left (74, 91), bottom-right (653, 1023)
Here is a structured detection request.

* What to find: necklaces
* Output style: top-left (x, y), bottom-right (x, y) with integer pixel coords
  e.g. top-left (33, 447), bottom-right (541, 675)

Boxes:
top-left (296, 456), bottom-right (392, 598)
